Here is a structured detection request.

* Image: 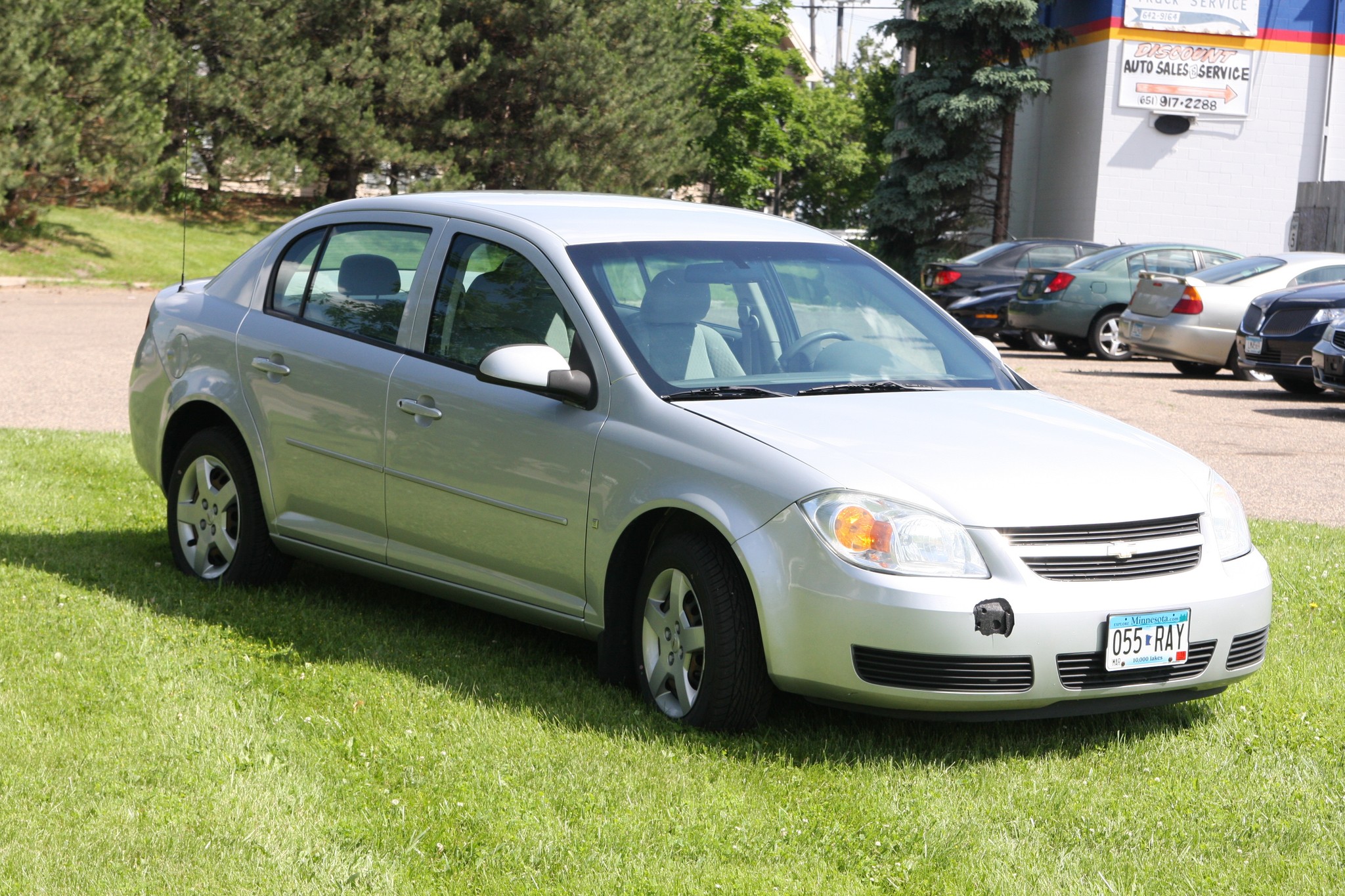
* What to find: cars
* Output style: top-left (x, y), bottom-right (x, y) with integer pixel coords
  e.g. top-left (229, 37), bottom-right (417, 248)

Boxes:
top-left (123, 187), bottom-right (1274, 739)
top-left (1235, 275), bottom-right (1345, 402)
top-left (919, 231), bottom-right (1112, 352)
top-left (1007, 241), bottom-right (1269, 362)
top-left (1116, 250), bottom-right (1345, 381)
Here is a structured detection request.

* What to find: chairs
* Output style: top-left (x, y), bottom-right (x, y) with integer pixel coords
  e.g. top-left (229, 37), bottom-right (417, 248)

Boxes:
top-left (626, 267), bottom-right (748, 383)
top-left (324, 253), bottom-right (406, 344)
top-left (449, 270), bottom-right (549, 365)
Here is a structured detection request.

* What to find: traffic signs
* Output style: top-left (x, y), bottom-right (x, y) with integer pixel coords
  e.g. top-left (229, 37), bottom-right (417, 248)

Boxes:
top-left (1117, 38), bottom-right (1256, 119)
